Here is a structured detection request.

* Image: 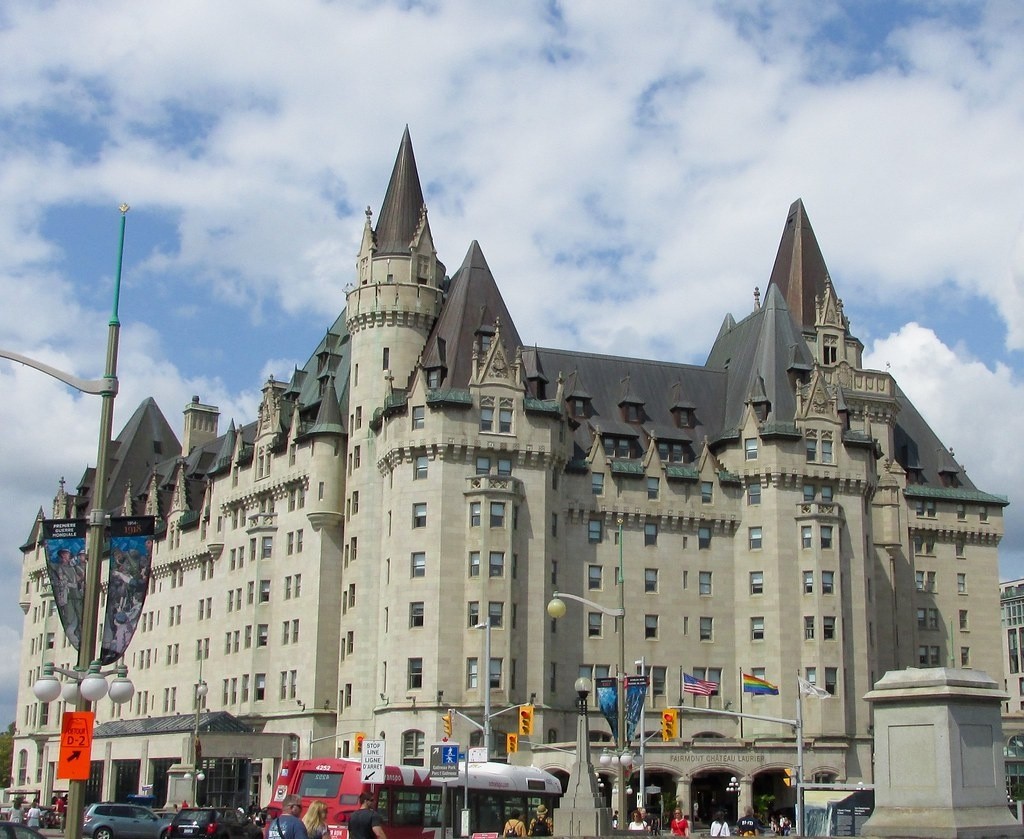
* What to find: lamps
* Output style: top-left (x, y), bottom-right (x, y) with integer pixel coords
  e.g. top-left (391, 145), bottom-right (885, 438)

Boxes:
top-left (120, 719), bottom-right (123, 720)
top-left (530, 692), bottom-right (536, 705)
top-left (437, 690), bottom-right (449, 706)
top-left (324, 700), bottom-right (330, 710)
top-left (267, 773), bottom-right (271, 786)
top-left (177, 712), bottom-right (180, 715)
top-left (725, 701), bottom-right (732, 710)
top-left (406, 696), bottom-right (416, 707)
top-left (13, 728), bottom-right (20, 734)
top-left (678, 698), bottom-right (684, 706)
top-left (380, 693), bottom-right (389, 705)
top-left (296, 700), bottom-right (305, 711)
top-left (96, 721), bottom-right (100, 725)
top-left (148, 715), bottom-right (151, 718)
top-left (206, 708), bottom-right (210, 712)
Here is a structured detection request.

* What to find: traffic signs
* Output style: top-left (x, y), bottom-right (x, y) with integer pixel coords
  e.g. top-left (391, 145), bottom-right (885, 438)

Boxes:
top-left (360, 739), bottom-right (386, 785)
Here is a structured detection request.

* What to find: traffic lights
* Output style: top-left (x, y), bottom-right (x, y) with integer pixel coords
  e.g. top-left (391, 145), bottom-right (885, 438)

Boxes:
top-left (507, 733), bottom-right (517, 753)
top-left (519, 705), bottom-right (534, 736)
top-left (661, 708), bottom-right (678, 742)
top-left (783, 768), bottom-right (797, 786)
top-left (442, 710), bottom-right (453, 737)
top-left (355, 731), bottom-right (366, 754)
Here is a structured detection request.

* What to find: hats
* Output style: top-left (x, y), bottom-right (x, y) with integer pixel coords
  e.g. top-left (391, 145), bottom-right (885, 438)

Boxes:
top-left (537, 805), bottom-right (548, 814)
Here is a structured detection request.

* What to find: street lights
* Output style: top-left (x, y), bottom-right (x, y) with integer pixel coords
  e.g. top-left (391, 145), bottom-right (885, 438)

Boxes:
top-left (546, 517), bottom-right (637, 831)
top-left (183, 653), bottom-right (209, 808)
top-left (473, 617), bottom-right (495, 763)
top-left (0, 201), bottom-right (137, 839)
top-left (725, 776), bottom-right (742, 825)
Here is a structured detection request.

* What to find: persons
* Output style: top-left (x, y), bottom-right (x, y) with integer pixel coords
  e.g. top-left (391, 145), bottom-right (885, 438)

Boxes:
top-left (737, 805), bottom-right (760, 837)
top-left (760, 815), bottom-right (792, 836)
top-left (238, 801), bottom-right (262, 827)
top-left (671, 807), bottom-right (692, 837)
top-left (528, 805), bottom-right (554, 837)
top-left (173, 804), bottom-right (178, 812)
top-left (302, 800), bottom-right (332, 839)
top-left (629, 809), bottom-right (661, 836)
top-left (27, 803), bottom-right (40, 832)
top-left (503, 809), bottom-right (527, 838)
top-left (56, 797), bottom-right (66, 833)
top-left (348, 791), bottom-right (387, 839)
top-left (266, 794), bottom-right (308, 839)
top-left (182, 800), bottom-right (189, 808)
top-left (8, 802), bottom-right (24, 824)
top-left (710, 811), bottom-right (730, 837)
top-left (613, 811), bottom-right (619, 829)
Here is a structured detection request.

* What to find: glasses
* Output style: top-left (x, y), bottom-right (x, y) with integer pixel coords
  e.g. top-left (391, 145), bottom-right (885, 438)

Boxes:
top-left (291, 803), bottom-right (302, 810)
top-left (367, 798), bottom-right (373, 802)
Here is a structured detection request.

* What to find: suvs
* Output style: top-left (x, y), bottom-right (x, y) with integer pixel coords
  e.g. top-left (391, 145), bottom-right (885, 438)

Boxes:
top-left (166, 806), bottom-right (265, 839)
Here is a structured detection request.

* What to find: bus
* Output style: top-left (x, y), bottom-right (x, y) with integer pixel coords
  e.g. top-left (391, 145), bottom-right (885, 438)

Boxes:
top-left (253, 757), bottom-right (563, 839)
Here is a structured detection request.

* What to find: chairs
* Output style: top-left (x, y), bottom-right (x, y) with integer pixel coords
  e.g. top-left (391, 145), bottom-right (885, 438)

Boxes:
top-left (226, 812), bottom-right (231, 818)
top-left (404, 810), bottom-right (437, 826)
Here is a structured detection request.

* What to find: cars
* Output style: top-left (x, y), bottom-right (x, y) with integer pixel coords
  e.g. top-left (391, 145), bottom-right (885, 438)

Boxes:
top-left (81, 800), bottom-right (173, 839)
top-left (256, 807), bottom-right (267, 826)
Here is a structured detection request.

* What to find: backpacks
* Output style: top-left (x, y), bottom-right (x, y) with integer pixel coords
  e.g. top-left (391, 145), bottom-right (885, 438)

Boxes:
top-left (506, 821), bottom-right (521, 837)
top-left (531, 816), bottom-right (552, 836)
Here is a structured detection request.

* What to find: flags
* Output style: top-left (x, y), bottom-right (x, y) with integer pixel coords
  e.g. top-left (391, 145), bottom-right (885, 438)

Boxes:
top-left (799, 676), bottom-right (832, 700)
top-left (682, 673), bottom-right (719, 696)
top-left (742, 672), bottom-right (779, 696)
